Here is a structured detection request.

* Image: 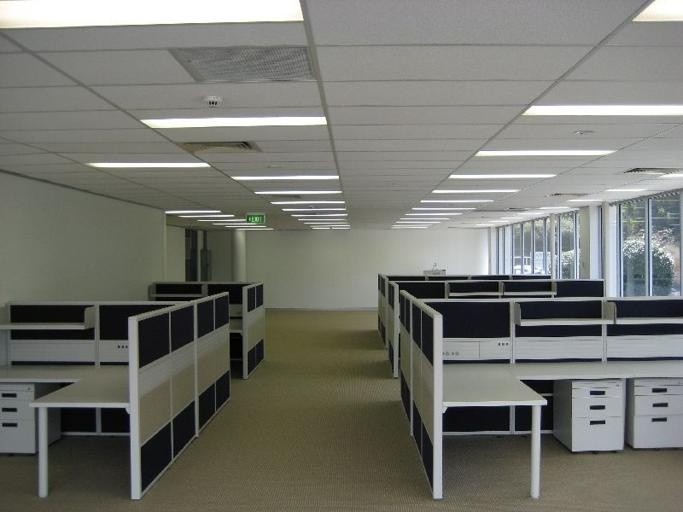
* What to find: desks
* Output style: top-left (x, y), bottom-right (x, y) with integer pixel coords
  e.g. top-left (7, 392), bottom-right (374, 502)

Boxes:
top-left (443, 359), bottom-right (683, 500)
top-left (0, 364), bottom-right (131, 498)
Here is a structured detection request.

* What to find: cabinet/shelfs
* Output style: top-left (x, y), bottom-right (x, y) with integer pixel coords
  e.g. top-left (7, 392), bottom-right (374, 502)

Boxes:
top-left (554, 378), bottom-right (683, 454)
top-left (0, 383), bottom-right (61, 456)
